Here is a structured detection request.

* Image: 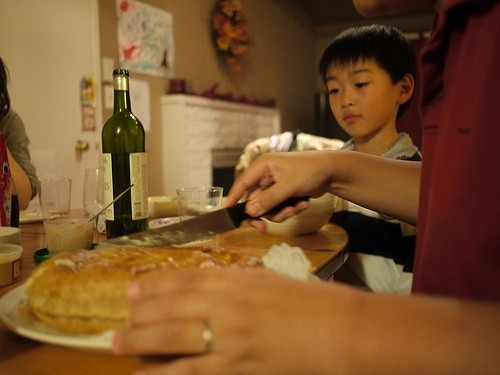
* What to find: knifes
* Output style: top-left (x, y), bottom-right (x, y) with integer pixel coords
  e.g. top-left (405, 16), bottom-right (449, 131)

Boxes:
top-left (104, 193), bottom-right (308, 245)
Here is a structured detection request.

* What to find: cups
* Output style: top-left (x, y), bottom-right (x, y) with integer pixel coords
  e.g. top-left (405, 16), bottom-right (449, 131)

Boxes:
top-left (44, 217), bottom-right (95, 259)
top-left (0, 227), bottom-right (20, 245)
top-left (82, 168), bottom-right (104, 217)
top-left (35, 177), bottom-right (72, 223)
top-left (176, 186), bottom-right (223, 222)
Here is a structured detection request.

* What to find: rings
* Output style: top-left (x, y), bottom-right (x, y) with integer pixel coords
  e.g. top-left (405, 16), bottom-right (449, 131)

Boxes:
top-left (200, 321), bottom-right (214, 355)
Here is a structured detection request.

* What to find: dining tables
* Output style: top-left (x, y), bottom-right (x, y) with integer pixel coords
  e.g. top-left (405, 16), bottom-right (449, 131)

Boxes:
top-left (0, 201), bottom-right (353, 375)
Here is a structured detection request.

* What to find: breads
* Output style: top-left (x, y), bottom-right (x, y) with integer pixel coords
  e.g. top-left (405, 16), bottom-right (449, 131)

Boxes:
top-left (25, 246), bottom-right (265, 337)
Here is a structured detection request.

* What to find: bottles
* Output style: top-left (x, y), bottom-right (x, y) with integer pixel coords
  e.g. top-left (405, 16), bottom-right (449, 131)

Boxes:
top-left (0, 243), bottom-right (24, 287)
top-left (101, 68), bottom-right (149, 239)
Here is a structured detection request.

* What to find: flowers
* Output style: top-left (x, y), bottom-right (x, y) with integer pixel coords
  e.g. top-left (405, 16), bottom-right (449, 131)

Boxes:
top-left (206, 0), bottom-right (255, 78)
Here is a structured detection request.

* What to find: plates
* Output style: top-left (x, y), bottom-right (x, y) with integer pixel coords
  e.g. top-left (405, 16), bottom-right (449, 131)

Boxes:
top-left (0, 282), bottom-right (114, 352)
top-left (149, 216), bottom-right (195, 230)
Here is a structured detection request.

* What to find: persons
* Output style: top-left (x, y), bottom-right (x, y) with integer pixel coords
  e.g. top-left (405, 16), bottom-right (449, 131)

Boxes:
top-left (245, 24), bottom-right (424, 298)
top-left (1, 57), bottom-right (38, 214)
top-left (112, 1), bottom-right (500, 374)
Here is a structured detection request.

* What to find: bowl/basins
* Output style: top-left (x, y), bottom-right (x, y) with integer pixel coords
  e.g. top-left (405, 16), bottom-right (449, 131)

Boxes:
top-left (148, 196), bottom-right (178, 219)
top-left (260, 192), bottom-right (335, 237)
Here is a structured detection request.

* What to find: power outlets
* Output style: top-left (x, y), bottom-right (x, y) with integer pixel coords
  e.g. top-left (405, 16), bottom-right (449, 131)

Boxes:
top-left (82, 105), bottom-right (97, 131)
